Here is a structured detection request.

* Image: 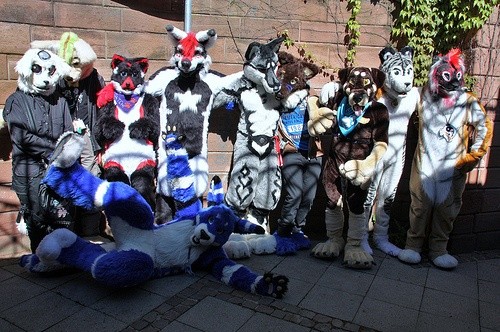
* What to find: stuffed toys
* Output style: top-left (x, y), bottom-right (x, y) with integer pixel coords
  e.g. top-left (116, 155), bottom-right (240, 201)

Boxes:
top-left (316, 47), bottom-right (423, 258)
top-left (303, 64), bottom-right (390, 268)
top-left (272, 51), bottom-right (323, 255)
top-left (210, 37), bottom-right (310, 226)
top-left (2, 48), bottom-right (76, 256)
top-left (374, 49), bottom-right (493, 270)
top-left (144, 26), bottom-right (231, 225)
top-left (17, 128), bottom-right (312, 298)
top-left (93, 54), bottom-right (162, 216)
top-left (30, 32), bottom-right (115, 241)
